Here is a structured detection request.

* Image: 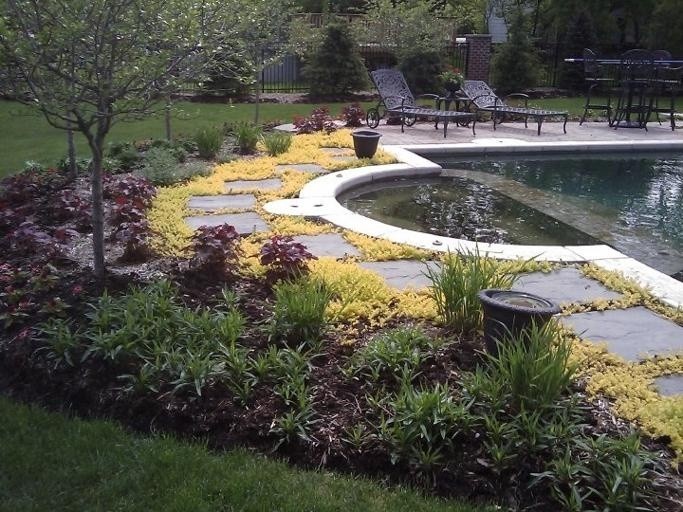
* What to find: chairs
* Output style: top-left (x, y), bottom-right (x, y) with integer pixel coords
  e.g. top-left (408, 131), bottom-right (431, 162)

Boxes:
top-left (365, 68), bottom-right (571, 140)
top-left (580, 48), bottom-right (682, 132)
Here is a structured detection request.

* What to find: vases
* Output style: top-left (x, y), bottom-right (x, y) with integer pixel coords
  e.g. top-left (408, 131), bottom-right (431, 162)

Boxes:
top-left (477, 285), bottom-right (560, 362)
top-left (349, 129), bottom-right (383, 159)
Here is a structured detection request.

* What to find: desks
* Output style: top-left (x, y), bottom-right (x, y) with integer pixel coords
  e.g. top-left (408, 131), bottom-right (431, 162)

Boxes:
top-left (562, 57), bottom-right (682, 129)
